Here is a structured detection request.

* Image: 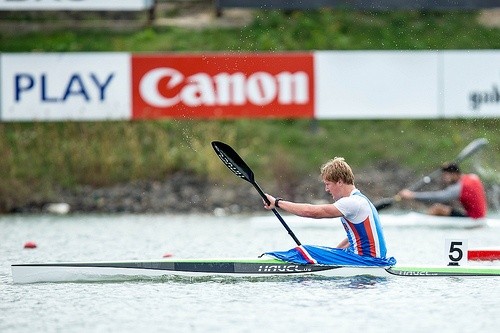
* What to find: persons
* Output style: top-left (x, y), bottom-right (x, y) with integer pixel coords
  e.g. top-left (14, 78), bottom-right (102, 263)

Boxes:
top-left (398, 160), bottom-right (487, 219)
top-left (258, 156), bottom-right (387, 266)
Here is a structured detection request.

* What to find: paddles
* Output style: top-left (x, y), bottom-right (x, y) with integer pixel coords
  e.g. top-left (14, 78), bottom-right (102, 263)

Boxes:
top-left (210, 140), bottom-right (318, 265)
top-left (370, 138), bottom-right (489, 210)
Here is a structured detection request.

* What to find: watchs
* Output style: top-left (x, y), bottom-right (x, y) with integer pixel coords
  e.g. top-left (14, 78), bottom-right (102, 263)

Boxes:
top-left (275, 197), bottom-right (284, 208)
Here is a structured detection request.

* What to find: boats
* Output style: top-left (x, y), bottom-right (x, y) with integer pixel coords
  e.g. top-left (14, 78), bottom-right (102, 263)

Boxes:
top-left (252, 212), bottom-right (499, 229)
top-left (8, 259), bottom-right (500, 287)
